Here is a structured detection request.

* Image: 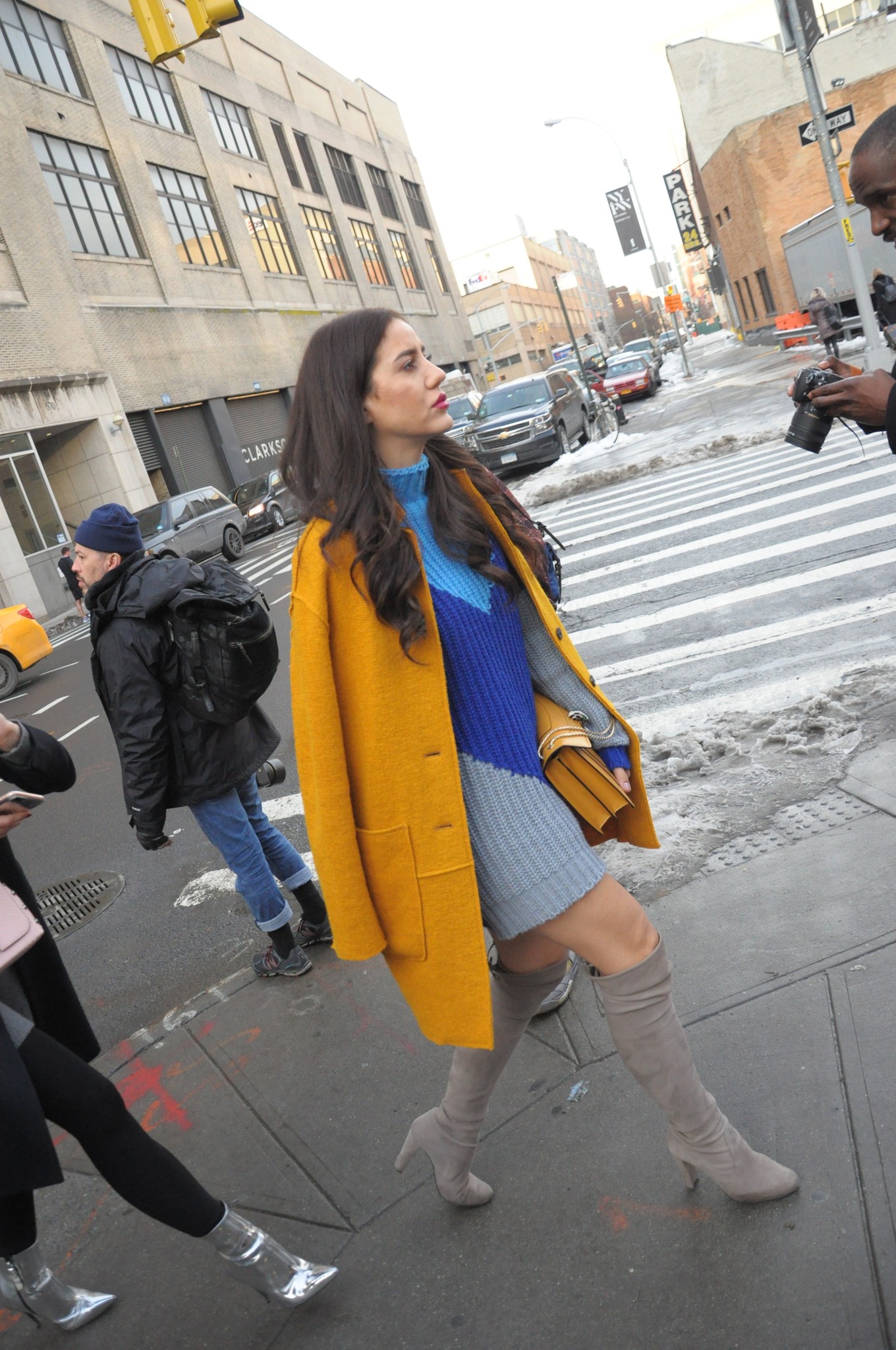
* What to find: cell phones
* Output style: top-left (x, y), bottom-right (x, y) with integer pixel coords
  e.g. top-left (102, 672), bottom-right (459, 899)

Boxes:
top-left (0, 791), bottom-right (44, 809)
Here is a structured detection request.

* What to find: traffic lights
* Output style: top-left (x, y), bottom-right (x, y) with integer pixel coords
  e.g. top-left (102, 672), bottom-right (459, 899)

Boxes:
top-left (500, 375), bottom-right (506, 381)
top-left (537, 324), bottom-right (543, 333)
top-left (184, 0), bottom-right (244, 41)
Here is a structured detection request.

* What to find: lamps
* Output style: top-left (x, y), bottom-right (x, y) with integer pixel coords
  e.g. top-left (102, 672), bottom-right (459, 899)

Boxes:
top-left (831, 77), bottom-right (844, 88)
top-left (110, 414), bottom-right (124, 436)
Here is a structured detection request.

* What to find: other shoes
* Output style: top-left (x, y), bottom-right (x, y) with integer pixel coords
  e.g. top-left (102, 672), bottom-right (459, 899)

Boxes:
top-left (83, 617), bottom-right (91, 622)
top-left (532, 950), bottom-right (582, 1019)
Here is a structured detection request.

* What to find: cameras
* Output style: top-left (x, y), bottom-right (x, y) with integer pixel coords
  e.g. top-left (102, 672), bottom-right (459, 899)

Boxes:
top-left (256, 758), bottom-right (286, 789)
top-left (784, 367), bottom-right (847, 454)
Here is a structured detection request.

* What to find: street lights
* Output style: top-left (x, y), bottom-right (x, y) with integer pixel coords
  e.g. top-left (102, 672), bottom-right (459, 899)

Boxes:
top-left (474, 283), bottom-right (511, 386)
top-left (544, 117), bottom-right (691, 376)
top-left (642, 311), bottom-right (655, 336)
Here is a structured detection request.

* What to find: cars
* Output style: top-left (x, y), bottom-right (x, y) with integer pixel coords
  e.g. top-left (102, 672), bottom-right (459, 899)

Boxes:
top-left (444, 389), bottom-right (483, 447)
top-left (132, 486), bottom-right (248, 563)
top-left (659, 327), bottom-right (691, 354)
top-left (547, 352), bottom-right (608, 382)
top-left (227, 467), bottom-right (300, 537)
top-left (0, 604), bottom-right (53, 699)
top-left (585, 336), bottom-right (662, 398)
top-left (462, 368), bottom-right (591, 471)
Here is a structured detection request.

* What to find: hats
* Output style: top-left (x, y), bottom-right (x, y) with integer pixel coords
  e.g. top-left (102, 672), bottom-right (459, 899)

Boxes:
top-left (74, 503), bottom-right (144, 556)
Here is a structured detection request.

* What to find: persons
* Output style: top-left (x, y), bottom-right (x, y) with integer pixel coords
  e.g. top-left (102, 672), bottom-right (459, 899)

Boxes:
top-left (71, 503), bottom-right (334, 978)
top-left (0, 712), bottom-right (341, 1332)
top-left (787, 105), bottom-right (896, 456)
top-left (872, 268), bottom-right (896, 346)
top-left (581, 356), bottom-right (597, 369)
top-left (808, 287), bottom-right (843, 359)
top-left (285, 307), bottom-right (798, 1209)
top-left (58, 547), bottom-right (92, 623)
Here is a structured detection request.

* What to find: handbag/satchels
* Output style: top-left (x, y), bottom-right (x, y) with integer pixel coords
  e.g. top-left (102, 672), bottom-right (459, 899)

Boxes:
top-left (532, 688), bottom-right (633, 837)
top-left (0, 881), bottom-right (45, 971)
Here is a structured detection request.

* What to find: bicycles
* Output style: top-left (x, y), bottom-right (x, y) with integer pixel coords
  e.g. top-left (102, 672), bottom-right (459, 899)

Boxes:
top-left (590, 390), bottom-right (619, 449)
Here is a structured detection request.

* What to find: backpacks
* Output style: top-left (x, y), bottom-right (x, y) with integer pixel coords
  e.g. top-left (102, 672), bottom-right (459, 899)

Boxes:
top-left (152, 558), bottom-right (281, 724)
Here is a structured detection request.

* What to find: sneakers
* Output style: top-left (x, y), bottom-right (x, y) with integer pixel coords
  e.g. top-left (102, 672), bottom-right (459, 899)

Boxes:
top-left (294, 910), bottom-right (333, 947)
top-left (252, 946), bottom-right (311, 977)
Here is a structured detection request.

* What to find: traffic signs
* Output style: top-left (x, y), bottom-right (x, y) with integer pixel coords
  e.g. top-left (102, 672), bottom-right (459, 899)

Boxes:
top-left (797, 102), bottom-right (856, 148)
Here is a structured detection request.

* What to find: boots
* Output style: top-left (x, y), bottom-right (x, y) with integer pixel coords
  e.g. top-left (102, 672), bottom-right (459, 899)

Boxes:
top-left (0, 1239), bottom-right (116, 1331)
top-left (586, 935), bottom-right (800, 1202)
top-left (202, 1201), bottom-right (338, 1308)
top-left (393, 958), bottom-right (570, 1208)
